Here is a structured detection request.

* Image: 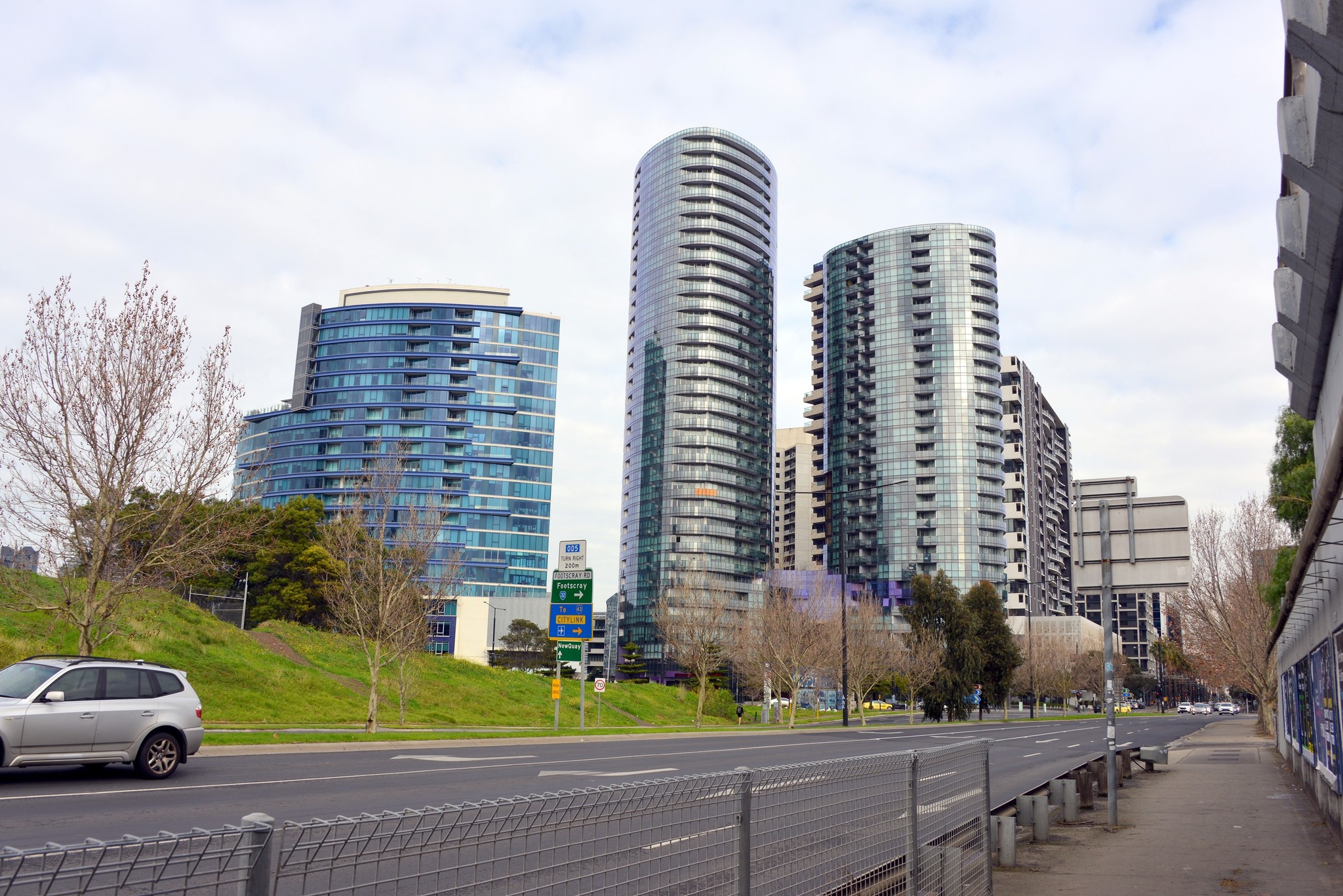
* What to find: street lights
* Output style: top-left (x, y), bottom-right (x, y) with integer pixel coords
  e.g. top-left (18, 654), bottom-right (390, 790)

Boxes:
top-left (775, 479), bottom-right (909, 728)
top-left (484, 601), bottom-right (506, 668)
top-left (988, 577), bottom-right (1070, 719)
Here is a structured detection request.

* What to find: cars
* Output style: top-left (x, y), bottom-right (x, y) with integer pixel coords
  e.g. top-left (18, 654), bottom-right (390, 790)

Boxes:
top-left (770, 697), bottom-right (790, 706)
top-left (1218, 703), bottom-right (1235, 715)
top-left (1177, 702), bottom-right (1193, 713)
top-left (1121, 699), bottom-right (1145, 709)
top-left (1232, 704), bottom-right (1241, 714)
top-left (913, 702), bottom-right (925, 710)
top-left (1213, 703), bottom-right (1221, 711)
top-left (1205, 704), bottom-right (1212, 714)
top-left (863, 699), bottom-right (893, 710)
top-left (1104, 704), bottom-right (1131, 714)
top-left (1192, 703), bottom-right (1207, 715)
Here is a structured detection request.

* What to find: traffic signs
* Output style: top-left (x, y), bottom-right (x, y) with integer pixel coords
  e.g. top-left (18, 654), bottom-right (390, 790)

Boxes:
top-left (550, 540), bottom-right (593, 661)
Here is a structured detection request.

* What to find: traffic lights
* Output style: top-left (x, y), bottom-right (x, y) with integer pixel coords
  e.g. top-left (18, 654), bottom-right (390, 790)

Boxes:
top-left (1076, 692), bottom-right (1080, 699)
top-left (1156, 692), bottom-right (1159, 698)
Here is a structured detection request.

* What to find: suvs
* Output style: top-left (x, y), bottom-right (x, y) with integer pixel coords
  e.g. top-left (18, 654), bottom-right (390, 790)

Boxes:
top-left (0, 653), bottom-right (204, 779)
top-left (1094, 703), bottom-right (1106, 713)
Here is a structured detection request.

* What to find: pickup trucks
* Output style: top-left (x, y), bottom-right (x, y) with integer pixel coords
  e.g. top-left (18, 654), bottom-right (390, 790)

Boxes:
top-left (882, 698), bottom-right (908, 709)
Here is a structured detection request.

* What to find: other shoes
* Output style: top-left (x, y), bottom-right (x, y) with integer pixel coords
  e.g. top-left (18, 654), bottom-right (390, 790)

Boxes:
top-left (738, 723), bottom-right (741, 725)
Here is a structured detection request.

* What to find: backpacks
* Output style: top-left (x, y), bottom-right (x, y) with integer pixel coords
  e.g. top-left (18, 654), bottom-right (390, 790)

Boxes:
top-left (737, 706), bottom-right (742, 713)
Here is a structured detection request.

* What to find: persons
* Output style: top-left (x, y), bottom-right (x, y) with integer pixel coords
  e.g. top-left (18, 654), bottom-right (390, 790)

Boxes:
top-left (736, 703), bottom-right (744, 725)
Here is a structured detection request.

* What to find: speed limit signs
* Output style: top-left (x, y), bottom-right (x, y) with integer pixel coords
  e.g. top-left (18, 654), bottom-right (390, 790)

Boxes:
top-left (594, 678), bottom-right (605, 692)
